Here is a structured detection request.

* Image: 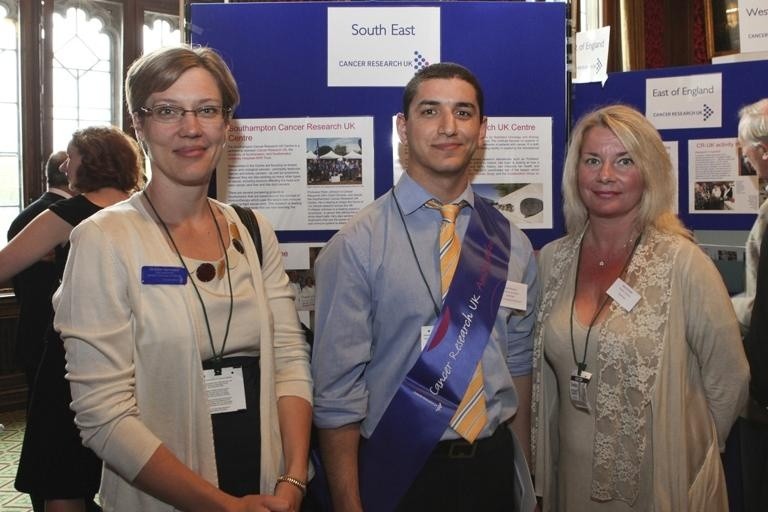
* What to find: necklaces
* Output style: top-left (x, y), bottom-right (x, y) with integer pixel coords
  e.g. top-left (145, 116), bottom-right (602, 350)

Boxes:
top-left (588, 230), bottom-right (639, 269)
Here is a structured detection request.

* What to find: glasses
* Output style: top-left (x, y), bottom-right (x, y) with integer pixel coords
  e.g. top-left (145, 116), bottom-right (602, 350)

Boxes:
top-left (138, 102), bottom-right (233, 122)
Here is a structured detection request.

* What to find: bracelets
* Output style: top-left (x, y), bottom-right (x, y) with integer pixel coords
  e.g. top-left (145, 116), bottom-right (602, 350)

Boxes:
top-left (273, 477), bottom-right (308, 498)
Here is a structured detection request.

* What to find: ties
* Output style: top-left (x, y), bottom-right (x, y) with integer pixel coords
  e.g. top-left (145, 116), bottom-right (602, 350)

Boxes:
top-left (422, 198), bottom-right (489, 446)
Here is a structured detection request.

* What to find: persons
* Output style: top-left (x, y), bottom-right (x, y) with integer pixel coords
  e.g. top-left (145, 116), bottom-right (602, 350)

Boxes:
top-left (53, 45), bottom-right (315, 512)
top-left (311, 62), bottom-right (542, 511)
top-left (0, 125), bottom-right (146, 512)
top-left (7, 152), bottom-right (72, 421)
top-left (531, 104), bottom-right (751, 511)
top-left (731, 96), bottom-right (767, 511)
